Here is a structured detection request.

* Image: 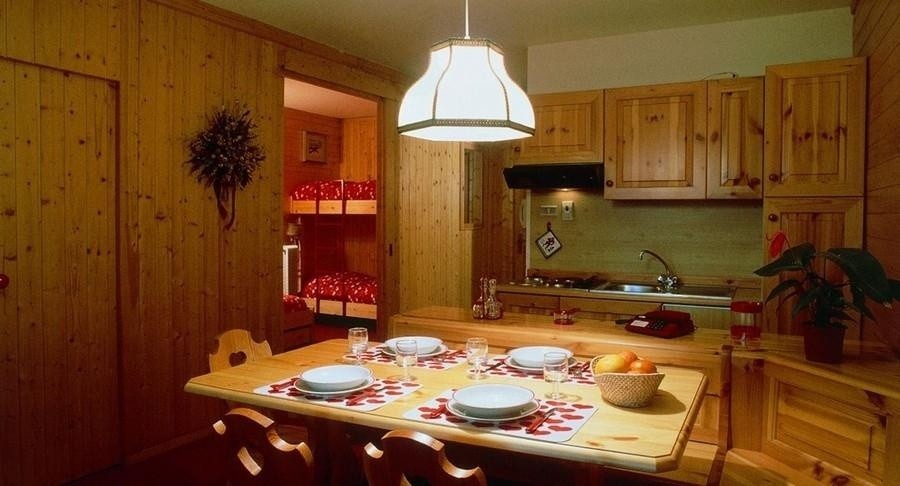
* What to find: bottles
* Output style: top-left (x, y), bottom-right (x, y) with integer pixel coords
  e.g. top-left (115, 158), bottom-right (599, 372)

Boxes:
top-left (472, 276), bottom-right (502, 319)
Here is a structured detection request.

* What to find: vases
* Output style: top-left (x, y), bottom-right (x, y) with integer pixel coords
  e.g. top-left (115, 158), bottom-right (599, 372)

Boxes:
top-left (800, 321), bottom-right (848, 362)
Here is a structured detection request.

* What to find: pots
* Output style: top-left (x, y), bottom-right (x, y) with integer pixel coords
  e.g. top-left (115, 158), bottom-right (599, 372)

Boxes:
top-left (553, 307), bottom-right (581, 325)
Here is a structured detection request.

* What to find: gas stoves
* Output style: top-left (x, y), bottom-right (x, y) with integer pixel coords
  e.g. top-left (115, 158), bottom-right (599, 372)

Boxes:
top-left (519, 271), bottom-right (605, 289)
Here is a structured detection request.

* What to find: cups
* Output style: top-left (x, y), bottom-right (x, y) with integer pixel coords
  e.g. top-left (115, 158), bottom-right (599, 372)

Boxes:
top-left (731, 301), bottom-right (763, 345)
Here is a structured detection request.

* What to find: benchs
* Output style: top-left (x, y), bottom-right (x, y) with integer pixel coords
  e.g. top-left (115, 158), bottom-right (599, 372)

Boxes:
top-left (386, 315), bottom-right (900, 485)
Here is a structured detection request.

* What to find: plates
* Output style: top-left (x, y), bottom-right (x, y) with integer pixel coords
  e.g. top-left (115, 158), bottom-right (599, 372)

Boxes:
top-left (504, 356), bottom-right (579, 373)
top-left (442, 400), bottom-right (544, 421)
top-left (291, 378), bottom-right (377, 397)
top-left (380, 345), bottom-right (450, 359)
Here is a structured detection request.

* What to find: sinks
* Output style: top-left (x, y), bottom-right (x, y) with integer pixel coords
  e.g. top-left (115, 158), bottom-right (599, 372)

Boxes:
top-left (600, 280), bottom-right (660, 295)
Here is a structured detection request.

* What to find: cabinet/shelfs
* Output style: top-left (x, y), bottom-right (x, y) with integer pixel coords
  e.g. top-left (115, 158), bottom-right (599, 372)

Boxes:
top-left (504, 88), bottom-right (605, 165)
top-left (764, 55), bottom-right (867, 195)
top-left (604, 75), bottom-right (763, 201)
top-left (763, 196), bottom-right (865, 338)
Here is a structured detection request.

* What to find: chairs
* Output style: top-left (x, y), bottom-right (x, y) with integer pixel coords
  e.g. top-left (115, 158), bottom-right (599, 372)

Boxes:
top-left (361, 427), bottom-right (486, 486)
top-left (205, 329), bottom-right (312, 452)
top-left (211, 407), bottom-right (318, 485)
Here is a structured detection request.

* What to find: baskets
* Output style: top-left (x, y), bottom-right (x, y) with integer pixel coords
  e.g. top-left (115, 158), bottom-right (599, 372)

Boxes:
top-left (591, 353), bottom-right (666, 409)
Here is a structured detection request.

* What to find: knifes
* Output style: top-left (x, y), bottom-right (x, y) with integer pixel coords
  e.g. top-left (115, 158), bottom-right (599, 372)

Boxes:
top-left (524, 406), bottom-right (566, 433)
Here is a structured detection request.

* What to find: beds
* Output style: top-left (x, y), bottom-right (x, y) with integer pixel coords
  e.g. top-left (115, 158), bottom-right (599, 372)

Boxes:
top-left (283, 177), bottom-right (380, 320)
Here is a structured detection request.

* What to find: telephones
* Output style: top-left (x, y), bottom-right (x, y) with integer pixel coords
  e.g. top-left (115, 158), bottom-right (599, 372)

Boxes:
top-left (624, 310), bottom-right (694, 338)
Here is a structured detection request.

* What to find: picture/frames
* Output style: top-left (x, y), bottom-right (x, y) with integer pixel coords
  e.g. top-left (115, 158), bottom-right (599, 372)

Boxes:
top-left (301, 129), bottom-right (329, 163)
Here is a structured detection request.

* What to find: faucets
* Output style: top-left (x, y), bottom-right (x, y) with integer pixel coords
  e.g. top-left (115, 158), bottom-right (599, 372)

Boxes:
top-left (637, 248), bottom-right (675, 290)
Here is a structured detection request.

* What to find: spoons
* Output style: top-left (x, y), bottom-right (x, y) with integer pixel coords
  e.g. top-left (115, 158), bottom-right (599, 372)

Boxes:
top-left (570, 360), bottom-right (589, 378)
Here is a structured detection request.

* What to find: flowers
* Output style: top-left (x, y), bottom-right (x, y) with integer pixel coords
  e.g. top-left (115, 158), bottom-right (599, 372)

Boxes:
top-left (754, 230), bottom-right (900, 329)
top-left (188, 101), bottom-right (268, 192)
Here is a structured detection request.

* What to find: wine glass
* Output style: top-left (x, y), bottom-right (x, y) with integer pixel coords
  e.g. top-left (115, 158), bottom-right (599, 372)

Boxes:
top-left (542, 351), bottom-right (570, 400)
top-left (395, 340), bottom-right (419, 381)
top-left (464, 337), bottom-right (490, 379)
top-left (347, 327), bottom-right (369, 364)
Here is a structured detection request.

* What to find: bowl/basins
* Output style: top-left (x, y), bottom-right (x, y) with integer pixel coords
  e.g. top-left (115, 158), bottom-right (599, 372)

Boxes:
top-left (455, 383), bottom-right (535, 409)
top-left (301, 365), bottom-right (373, 385)
top-left (508, 346), bottom-right (574, 366)
top-left (384, 336), bottom-right (444, 354)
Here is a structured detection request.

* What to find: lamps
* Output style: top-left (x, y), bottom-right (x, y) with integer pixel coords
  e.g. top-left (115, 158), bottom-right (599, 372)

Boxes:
top-left (285, 220), bottom-right (306, 247)
top-left (395, 0), bottom-right (538, 148)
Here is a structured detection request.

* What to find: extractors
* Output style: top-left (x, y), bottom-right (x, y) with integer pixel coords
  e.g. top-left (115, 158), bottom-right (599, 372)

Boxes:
top-left (503, 163), bottom-right (602, 189)
